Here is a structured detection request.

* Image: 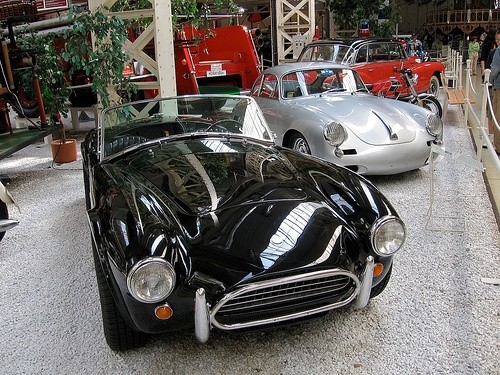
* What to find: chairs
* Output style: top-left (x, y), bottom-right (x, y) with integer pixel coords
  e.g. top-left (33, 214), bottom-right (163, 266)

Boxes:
top-left (104, 136), bottom-right (146, 157)
top-left (293, 86), bottom-right (326, 97)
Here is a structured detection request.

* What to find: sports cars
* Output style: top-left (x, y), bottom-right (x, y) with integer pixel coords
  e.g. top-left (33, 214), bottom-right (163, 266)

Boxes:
top-left (81, 92), bottom-right (406, 351)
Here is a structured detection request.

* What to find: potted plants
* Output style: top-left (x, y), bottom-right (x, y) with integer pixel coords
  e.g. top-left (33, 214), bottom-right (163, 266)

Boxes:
top-left (13, 5), bottom-right (137, 163)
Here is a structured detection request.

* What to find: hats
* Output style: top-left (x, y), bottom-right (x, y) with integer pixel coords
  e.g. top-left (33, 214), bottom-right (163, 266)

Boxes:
top-left (412, 32), bottom-right (417, 37)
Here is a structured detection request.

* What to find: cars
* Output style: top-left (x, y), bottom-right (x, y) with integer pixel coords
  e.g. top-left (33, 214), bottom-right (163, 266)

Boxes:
top-left (245, 36), bottom-right (448, 176)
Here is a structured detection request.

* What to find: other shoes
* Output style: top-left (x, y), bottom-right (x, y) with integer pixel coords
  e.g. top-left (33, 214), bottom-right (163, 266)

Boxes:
top-left (482, 143), bottom-right (487, 148)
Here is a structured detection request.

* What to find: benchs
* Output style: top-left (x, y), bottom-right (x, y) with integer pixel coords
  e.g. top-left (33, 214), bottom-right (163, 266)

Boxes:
top-left (440, 73), bottom-right (476, 122)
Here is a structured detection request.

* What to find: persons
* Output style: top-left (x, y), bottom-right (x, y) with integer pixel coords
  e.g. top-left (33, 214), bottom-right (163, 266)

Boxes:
top-left (480, 27), bottom-right (500, 83)
top-left (489, 30), bottom-right (500, 155)
top-left (423, 40), bottom-right (426, 48)
top-left (468, 37), bottom-right (479, 76)
top-left (432, 35), bottom-right (443, 50)
top-left (407, 33), bottom-right (422, 47)
